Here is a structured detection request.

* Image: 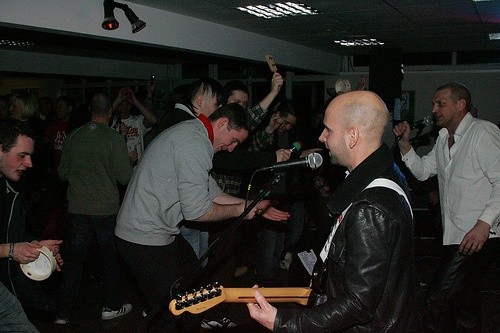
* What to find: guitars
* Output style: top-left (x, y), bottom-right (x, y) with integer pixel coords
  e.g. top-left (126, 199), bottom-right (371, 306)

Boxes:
top-left (168, 266), bottom-right (327, 316)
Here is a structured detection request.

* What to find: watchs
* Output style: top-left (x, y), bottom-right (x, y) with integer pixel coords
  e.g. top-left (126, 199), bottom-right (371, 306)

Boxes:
top-left (7, 241), bottom-right (15, 261)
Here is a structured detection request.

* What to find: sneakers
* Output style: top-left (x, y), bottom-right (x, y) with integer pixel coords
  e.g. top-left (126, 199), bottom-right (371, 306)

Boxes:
top-left (201, 317), bottom-right (238, 328)
top-left (101, 303), bottom-right (133, 320)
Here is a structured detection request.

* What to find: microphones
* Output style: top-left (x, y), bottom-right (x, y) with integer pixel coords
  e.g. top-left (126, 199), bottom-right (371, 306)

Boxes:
top-left (410, 115), bottom-right (434, 130)
top-left (255, 152), bottom-right (323, 176)
top-left (289, 140), bottom-right (303, 152)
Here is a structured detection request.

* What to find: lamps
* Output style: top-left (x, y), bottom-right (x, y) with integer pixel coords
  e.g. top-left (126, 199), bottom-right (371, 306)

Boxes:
top-left (101, 0), bottom-right (147, 35)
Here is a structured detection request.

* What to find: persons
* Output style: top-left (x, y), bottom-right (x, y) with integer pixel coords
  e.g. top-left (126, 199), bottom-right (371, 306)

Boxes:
top-left (155, 77), bottom-right (254, 330)
top-left (51, 96), bottom-right (133, 325)
top-left (242, 97), bottom-right (298, 288)
top-left (391, 84), bottom-right (499, 333)
top-left (117, 103), bottom-right (289, 332)
top-left (4, 91), bottom-right (56, 241)
top-left (107, 87), bottom-right (159, 171)
top-left (0, 119), bottom-right (64, 332)
top-left (247, 90), bottom-right (416, 333)
top-left (212, 71), bottom-right (284, 295)
